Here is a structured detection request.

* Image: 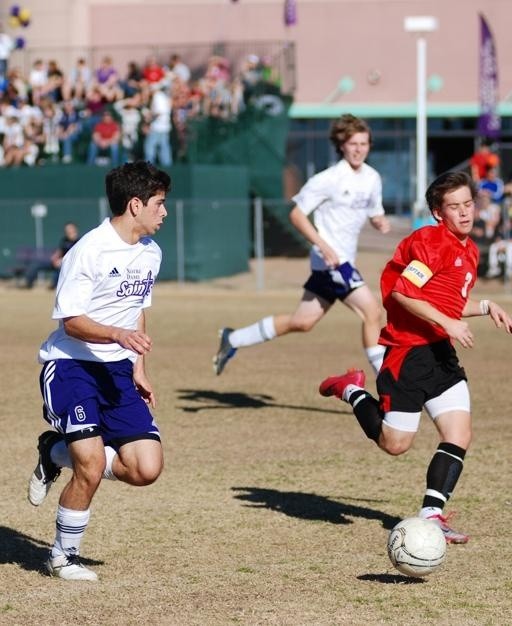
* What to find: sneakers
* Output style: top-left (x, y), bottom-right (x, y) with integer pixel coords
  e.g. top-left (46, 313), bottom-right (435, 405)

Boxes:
top-left (28, 430), bottom-right (63, 506)
top-left (213, 327), bottom-right (237, 375)
top-left (319, 370), bottom-right (365, 398)
top-left (46, 552), bottom-right (98, 580)
top-left (426, 512), bottom-right (467, 544)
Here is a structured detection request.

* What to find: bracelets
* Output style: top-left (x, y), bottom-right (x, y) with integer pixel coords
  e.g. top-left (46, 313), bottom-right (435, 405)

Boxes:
top-left (480, 299), bottom-right (490, 315)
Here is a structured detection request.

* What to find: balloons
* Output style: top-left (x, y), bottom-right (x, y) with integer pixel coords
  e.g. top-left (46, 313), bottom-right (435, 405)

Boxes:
top-left (7, 5), bottom-right (30, 28)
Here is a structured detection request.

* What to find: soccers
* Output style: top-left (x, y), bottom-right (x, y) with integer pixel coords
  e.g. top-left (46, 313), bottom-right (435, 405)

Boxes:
top-left (388, 517), bottom-right (447, 577)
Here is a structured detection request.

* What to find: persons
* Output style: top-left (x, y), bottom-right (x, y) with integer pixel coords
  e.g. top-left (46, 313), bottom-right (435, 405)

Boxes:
top-left (0, 32), bottom-right (271, 168)
top-left (25, 161), bottom-right (163, 581)
top-left (319, 171), bottom-right (512, 544)
top-left (21, 224), bottom-right (80, 289)
top-left (412, 138), bottom-right (512, 282)
top-left (212, 114), bottom-right (386, 377)
